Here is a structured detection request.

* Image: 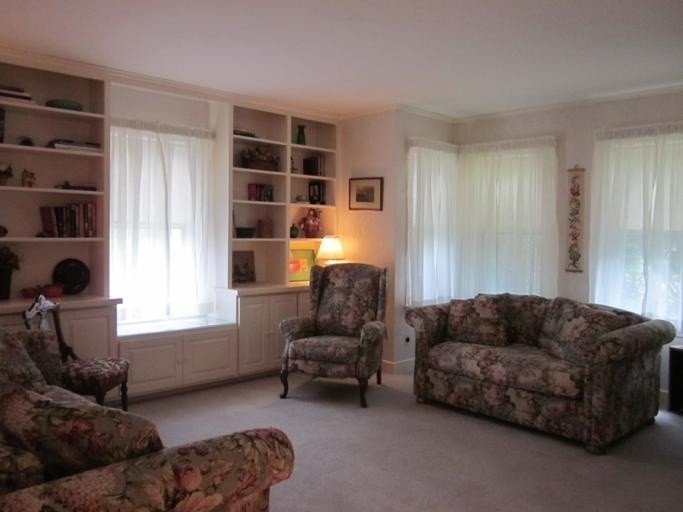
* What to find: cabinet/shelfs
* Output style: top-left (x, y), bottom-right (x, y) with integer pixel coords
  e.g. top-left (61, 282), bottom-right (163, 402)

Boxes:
top-left (227, 102), bottom-right (342, 383)
top-left (669, 345), bottom-right (683, 417)
top-left (118, 323), bottom-right (239, 403)
top-left (0, 42), bottom-right (123, 404)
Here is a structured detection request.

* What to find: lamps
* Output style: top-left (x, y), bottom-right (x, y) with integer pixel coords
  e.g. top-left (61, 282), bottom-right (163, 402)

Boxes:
top-left (315, 236), bottom-right (345, 267)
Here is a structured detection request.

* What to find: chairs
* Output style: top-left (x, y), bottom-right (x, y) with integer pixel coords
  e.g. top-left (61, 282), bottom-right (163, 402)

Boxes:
top-left (22, 294), bottom-right (130, 412)
top-left (1, 327), bottom-right (294, 510)
top-left (278, 263), bottom-right (388, 407)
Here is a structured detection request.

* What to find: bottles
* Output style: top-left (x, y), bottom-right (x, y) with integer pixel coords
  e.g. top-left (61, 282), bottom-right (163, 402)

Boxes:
top-left (291, 224), bottom-right (299, 238)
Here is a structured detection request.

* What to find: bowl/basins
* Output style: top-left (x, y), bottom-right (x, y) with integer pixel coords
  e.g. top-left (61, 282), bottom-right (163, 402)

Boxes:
top-left (237, 228), bottom-right (256, 237)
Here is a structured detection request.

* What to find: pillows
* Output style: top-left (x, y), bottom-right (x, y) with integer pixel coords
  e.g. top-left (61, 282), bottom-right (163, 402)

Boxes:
top-left (539, 296), bottom-right (644, 351)
top-left (549, 304), bottom-right (627, 370)
top-left (475, 294), bottom-right (548, 347)
top-left (447, 298), bottom-right (509, 347)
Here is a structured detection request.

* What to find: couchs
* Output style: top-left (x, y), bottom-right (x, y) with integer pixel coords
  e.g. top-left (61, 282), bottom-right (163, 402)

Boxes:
top-left (404, 296), bottom-right (677, 455)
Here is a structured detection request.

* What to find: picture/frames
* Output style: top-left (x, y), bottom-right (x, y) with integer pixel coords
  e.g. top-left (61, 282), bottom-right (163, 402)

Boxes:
top-left (349, 176), bottom-right (384, 210)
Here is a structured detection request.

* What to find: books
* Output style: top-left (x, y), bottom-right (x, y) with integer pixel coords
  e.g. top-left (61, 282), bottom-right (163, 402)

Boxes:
top-left (39, 201), bottom-right (98, 237)
top-left (303, 154), bottom-right (326, 177)
top-left (308, 181), bottom-right (327, 205)
top-left (248, 182), bottom-right (274, 201)
top-left (0, 86), bottom-right (38, 104)
